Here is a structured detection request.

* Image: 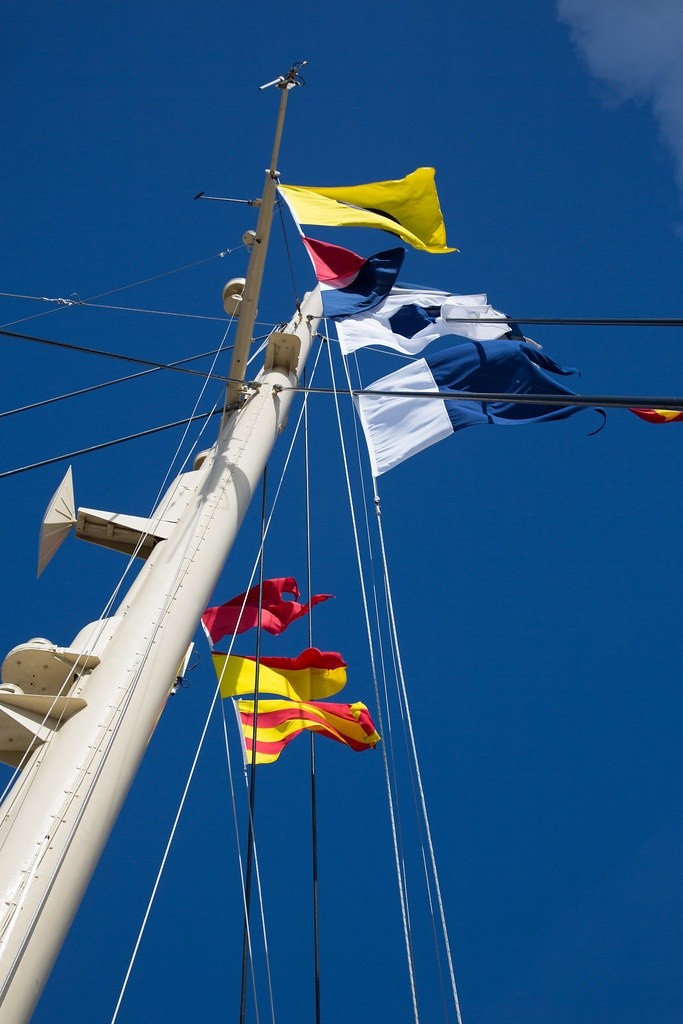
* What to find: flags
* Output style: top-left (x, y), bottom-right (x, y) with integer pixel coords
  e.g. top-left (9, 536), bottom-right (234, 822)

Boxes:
top-left (200, 576), bottom-right (337, 648)
top-left (298, 232), bottom-right (409, 320)
top-left (209, 649), bottom-right (347, 702)
top-left (351, 343), bottom-right (608, 478)
top-left (272, 165), bottom-right (456, 254)
top-left (330, 279), bottom-right (544, 356)
top-left (627, 407), bottom-right (681, 425)
top-left (229, 699), bottom-right (381, 766)
